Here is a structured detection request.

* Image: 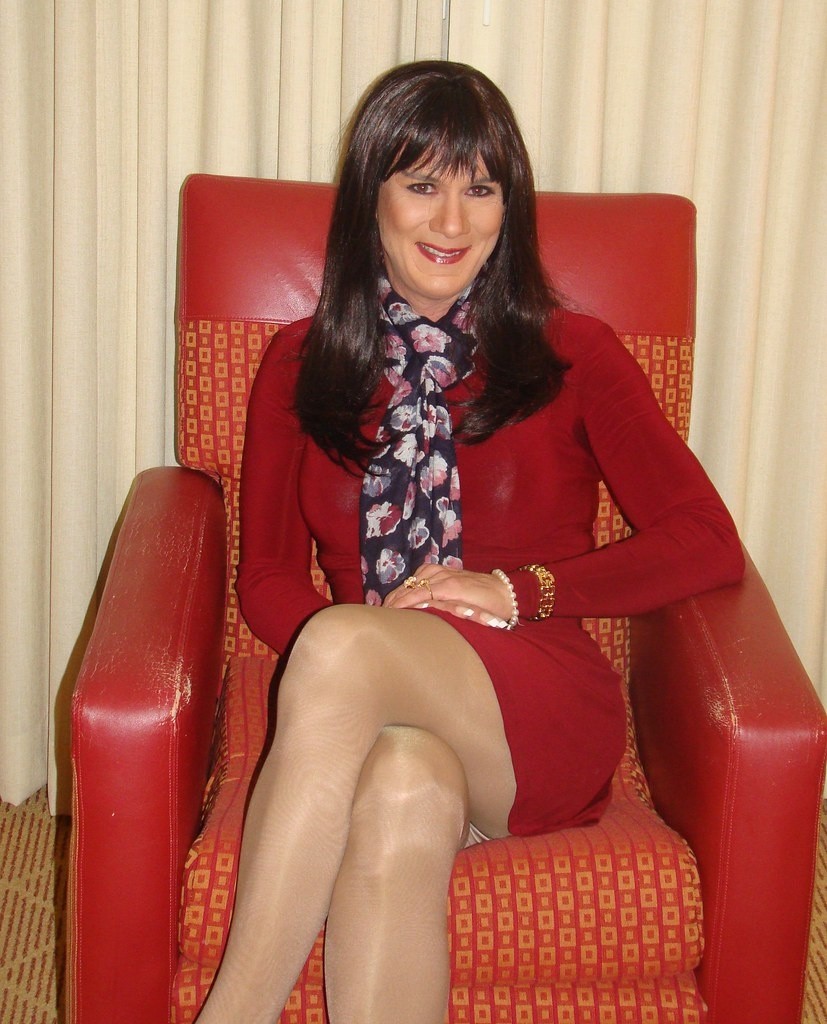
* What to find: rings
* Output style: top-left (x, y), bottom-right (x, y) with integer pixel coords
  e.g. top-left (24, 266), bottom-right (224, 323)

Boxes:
top-left (416, 579), bottom-right (434, 600)
top-left (403, 577), bottom-right (416, 589)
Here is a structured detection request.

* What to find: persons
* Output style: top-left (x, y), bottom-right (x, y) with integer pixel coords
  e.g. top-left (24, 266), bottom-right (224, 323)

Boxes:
top-left (190, 60), bottom-right (747, 1024)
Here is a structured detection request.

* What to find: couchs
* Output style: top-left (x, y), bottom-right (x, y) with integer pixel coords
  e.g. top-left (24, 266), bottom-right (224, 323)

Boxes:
top-left (65, 172), bottom-right (827, 1024)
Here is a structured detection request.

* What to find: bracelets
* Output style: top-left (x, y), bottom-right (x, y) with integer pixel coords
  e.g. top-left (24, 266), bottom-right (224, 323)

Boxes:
top-left (491, 569), bottom-right (520, 627)
top-left (516, 564), bottom-right (556, 621)
